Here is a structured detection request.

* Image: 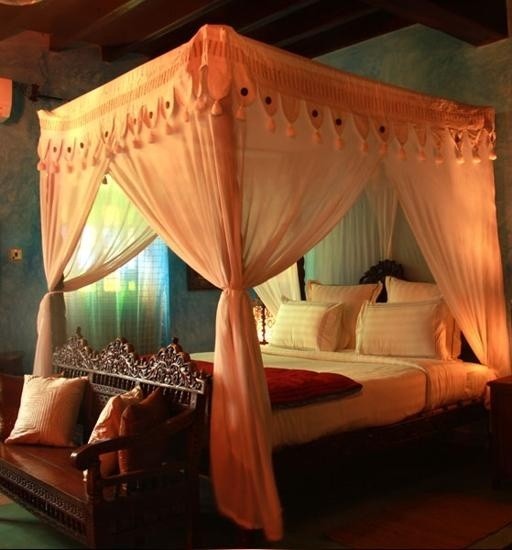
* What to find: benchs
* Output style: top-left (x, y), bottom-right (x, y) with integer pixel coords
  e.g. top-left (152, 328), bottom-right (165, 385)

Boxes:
top-left (0, 325), bottom-right (214, 550)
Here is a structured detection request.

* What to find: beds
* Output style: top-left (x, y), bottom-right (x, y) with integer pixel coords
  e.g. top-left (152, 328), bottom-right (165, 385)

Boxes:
top-left (40, 259), bottom-right (496, 548)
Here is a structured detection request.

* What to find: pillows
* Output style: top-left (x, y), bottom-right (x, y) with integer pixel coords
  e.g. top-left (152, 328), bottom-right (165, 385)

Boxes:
top-left (5, 371), bottom-right (167, 490)
top-left (265, 274), bottom-right (463, 361)
